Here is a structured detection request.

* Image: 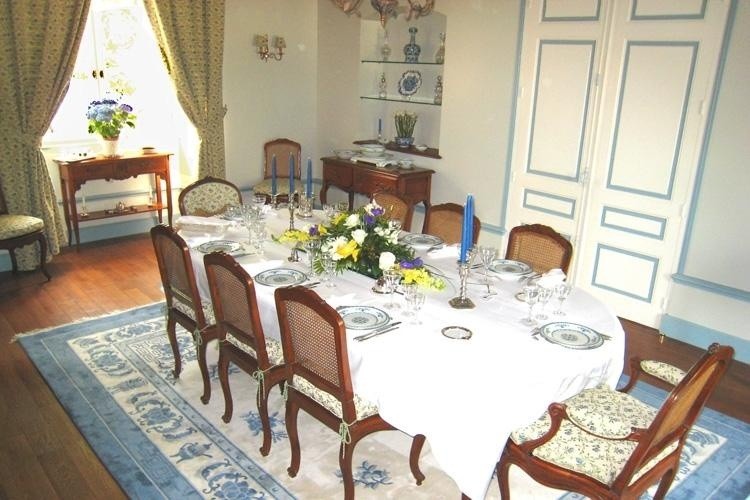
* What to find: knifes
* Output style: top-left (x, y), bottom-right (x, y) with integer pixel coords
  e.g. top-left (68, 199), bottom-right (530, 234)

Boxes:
top-left (304, 281), bottom-right (321, 289)
top-left (470, 262), bottom-right (483, 270)
top-left (354, 322), bottom-right (402, 343)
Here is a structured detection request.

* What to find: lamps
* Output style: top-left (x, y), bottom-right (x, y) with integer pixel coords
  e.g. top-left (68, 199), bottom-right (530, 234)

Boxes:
top-left (252, 33), bottom-right (287, 63)
top-left (330, 0), bottom-right (437, 28)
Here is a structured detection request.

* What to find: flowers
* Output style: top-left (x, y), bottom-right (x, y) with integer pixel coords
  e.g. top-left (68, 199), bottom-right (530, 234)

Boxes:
top-left (84, 99), bottom-right (138, 140)
top-left (269, 200), bottom-right (448, 292)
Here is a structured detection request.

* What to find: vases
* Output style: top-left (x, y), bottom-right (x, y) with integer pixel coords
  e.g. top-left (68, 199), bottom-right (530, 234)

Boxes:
top-left (101, 141), bottom-right (119, 158)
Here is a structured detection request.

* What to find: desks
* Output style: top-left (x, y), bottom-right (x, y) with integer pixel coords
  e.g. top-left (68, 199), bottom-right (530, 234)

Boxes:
top-left (319, 156), bottom-right (436, 232)
top-left (51, 152), bottom-right (176, 254)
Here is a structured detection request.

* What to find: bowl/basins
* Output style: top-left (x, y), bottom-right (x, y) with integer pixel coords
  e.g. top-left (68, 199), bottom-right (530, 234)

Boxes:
top-left (360, 144), bottom-right (385, 156)
top-left (334, 149), bottom-right (356, 159)
top-left (415, 145), bottom-right (427, 152)
top-left (397, 159), bottom-right (416, 169)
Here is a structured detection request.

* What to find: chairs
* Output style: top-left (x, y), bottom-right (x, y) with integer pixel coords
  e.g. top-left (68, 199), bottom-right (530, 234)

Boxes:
top-left (272, 283), bottom-right (426, 500)
top-left (368, 188), bottom-right (416, 233)
top-left (503, 223), bottom-right (574, 283)
top-left (1, 188), bottom-right (53, 283)
top-left (202, 250), bottom-right (286, 458)
top-left (494, 341), bottom-right (736, 499)
top-left (419, 202), bottom-right (483, 245)
top-left (252, 137), bottom-right (304, 205)
top-left (177, 175), bottom-right (244, 218)
top-left (149, 223), bottom-right (217, 406)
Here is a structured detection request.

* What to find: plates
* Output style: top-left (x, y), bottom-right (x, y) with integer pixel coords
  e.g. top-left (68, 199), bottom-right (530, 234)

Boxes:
top-left (488, 259), bottom-right (533, 275)
top-left (254, 268), bottom-right (307, 286)
top-left (336, 306), bottom-right (390, 330)
top-left (398, 71), bottom-right (422, 95)
top-left (198, 240), bottom-right (242, 254)
top-left (540, 321), bottom-right (604, 350)
top-left (402, 233), bottom-right (444, 246)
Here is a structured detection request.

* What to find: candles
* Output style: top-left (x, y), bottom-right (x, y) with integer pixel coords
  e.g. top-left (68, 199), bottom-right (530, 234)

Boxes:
top-left (306, 157), bottom-right (313, 200)
top-left (459, 194), bottom-right (476, 264)
top-left (271, 153), bottom-right (278, 196)
top-left (289, 153), bottom-right (296, 196)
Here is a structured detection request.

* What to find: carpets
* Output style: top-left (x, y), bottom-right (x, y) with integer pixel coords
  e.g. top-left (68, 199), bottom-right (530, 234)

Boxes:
top-left (11, 300), bottom-right (750, 500)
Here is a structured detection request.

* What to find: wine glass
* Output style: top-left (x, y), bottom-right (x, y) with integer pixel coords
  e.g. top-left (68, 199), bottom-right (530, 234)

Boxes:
top-left (383, 269), bottom-right (425, 325)
top-left (304, 239), bottom-right (336, 288)
top-left (457, 244), bottom-right (497, 284)
top-left (522, 285), bottom-right (537, 325)
top-left (224, 194), bottom-right (268, 253)
top-left (553, 284), bottom-right (571, 316)
top-left (323, 201), bottom-right (350, 225)
top-left (536, 287), bottom-right (554, 320)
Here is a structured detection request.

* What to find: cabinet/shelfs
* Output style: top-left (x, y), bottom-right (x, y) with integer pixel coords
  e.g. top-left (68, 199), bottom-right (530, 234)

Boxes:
top-left (352, 59), bottom-right (444, 161)
